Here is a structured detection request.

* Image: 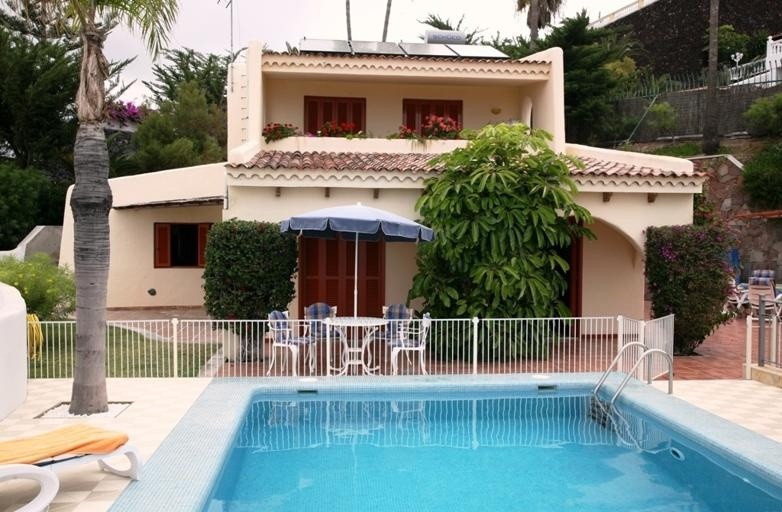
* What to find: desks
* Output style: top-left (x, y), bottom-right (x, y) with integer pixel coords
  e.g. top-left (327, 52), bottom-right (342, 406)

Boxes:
top-left (320, 315), bottom-right (385, 376)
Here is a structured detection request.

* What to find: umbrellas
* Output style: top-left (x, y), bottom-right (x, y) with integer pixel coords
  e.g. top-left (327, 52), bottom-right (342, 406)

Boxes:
top-left (279, 201), bottom-right (432, 317)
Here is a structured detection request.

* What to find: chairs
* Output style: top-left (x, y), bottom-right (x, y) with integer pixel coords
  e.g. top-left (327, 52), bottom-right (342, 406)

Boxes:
top-left (364, 303), bottom-right (414, 367)
top-left (303, 303), bottom-right (338, 374)
top-left (387, 312), bottom-right (431, 375)
top-left (265, 309), bottom-right (314, 377)
top-left (719, 270), bottom-right (782, 327)
